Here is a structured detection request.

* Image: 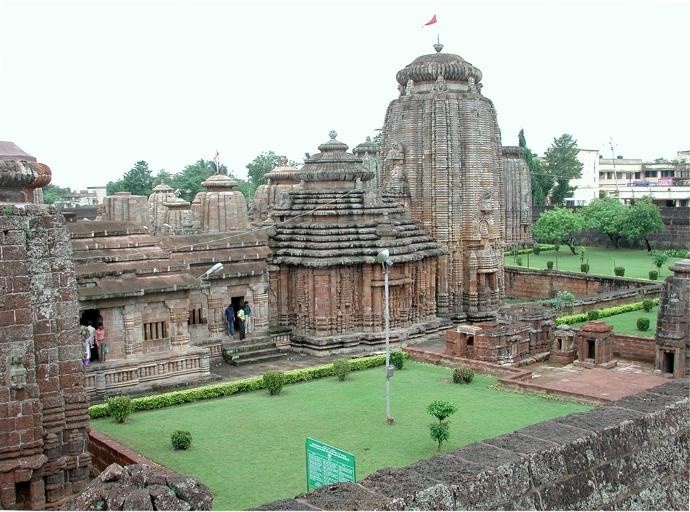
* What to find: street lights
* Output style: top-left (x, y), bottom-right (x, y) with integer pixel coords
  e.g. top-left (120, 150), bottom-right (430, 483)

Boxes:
top-left (375, 247), bottom-right (393, 426)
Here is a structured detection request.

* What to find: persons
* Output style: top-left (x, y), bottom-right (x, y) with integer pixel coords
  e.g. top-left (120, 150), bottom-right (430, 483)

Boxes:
top-left (95, 322), bottom-right (106, 362)
top-left (87, 320), bottom-right (96, 362)
top-left (225, 305), bottom-right (235, 335)
top-left (80, 317), bottom-right (91, 366)
top-left (237, 307), bottom-right (246, 340)
top-left (243, 301), bottom-right (251, 334)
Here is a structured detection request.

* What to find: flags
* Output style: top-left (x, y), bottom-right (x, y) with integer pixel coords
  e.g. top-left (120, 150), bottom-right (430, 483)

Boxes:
top-left (425, 13), bottom-right (437, 26)
